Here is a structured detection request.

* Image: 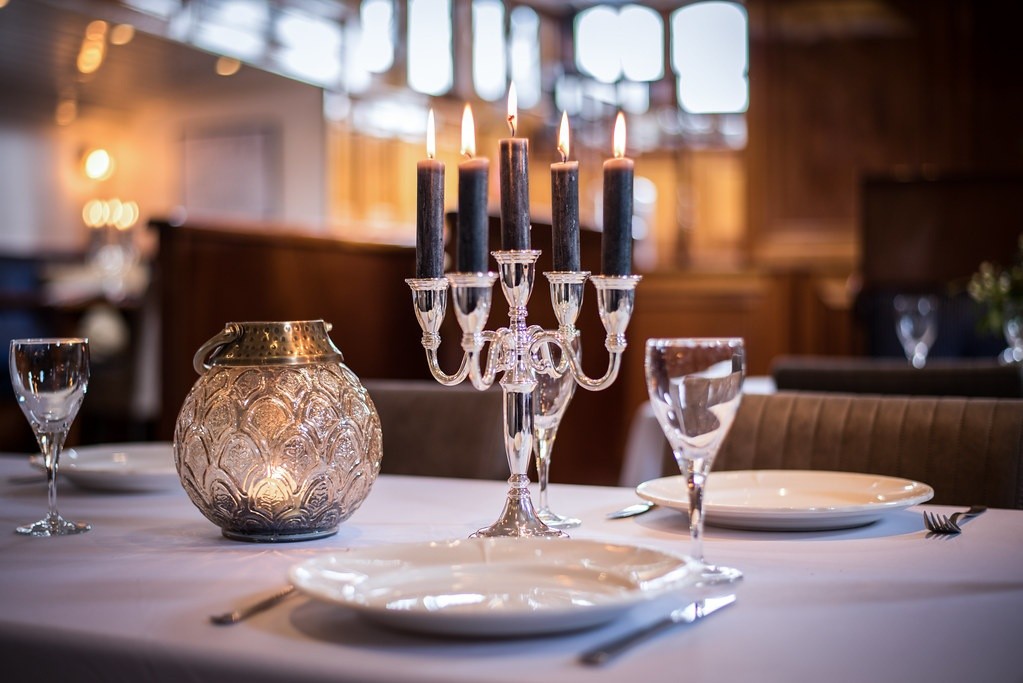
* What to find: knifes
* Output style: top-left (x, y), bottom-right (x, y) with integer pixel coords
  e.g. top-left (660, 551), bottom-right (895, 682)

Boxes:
top-left (579, 592), bottom-right (739, 663)
top-left (605, 499), bottom-right (655, 519)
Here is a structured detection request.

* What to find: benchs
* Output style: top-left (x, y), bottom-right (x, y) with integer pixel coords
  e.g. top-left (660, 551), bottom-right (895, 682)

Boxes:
top-left (767, 354), bottom-right (1023, 396)
top-left (352, 383), bottom-right (515, 479)
top-left (658, 392), bottom-right (1023, 510)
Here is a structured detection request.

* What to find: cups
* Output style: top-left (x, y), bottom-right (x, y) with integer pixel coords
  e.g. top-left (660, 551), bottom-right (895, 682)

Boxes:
top-left (896, 298), bottom-right (938, 367)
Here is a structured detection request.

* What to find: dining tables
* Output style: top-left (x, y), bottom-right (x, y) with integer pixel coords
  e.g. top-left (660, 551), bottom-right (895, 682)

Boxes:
top-left (0, 451), bottom-right (1023, 683)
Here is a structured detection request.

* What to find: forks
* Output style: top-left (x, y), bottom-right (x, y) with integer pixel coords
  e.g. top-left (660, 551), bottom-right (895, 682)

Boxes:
top-left (923, 505), bottom-right (987, 534)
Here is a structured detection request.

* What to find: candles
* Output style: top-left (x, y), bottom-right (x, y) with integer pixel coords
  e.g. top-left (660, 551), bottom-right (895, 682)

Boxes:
top-left (415, 109), bottom-right (446, 315)
top-left (600, 106), bottom-right (634, 326)
top-left (549, 109), bottom-right (585, 307)
top-left (457, 104), bottom-right (490, 316)
top-left (498, 76), bottom-right (532, 295)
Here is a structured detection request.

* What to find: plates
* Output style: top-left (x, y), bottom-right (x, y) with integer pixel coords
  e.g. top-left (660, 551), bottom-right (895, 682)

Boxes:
top-left (636, 469), bottom-right (935, 532)
top-left (286, 537), bottom-right (704, 637)
top-left (29, 444), bottom-right (181, 493)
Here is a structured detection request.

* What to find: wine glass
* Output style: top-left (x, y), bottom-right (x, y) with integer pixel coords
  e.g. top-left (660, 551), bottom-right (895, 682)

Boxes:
top-left (645, 337), bottom-right (748, 584)
top-left (9, 336), bottom-right (92, 536)
